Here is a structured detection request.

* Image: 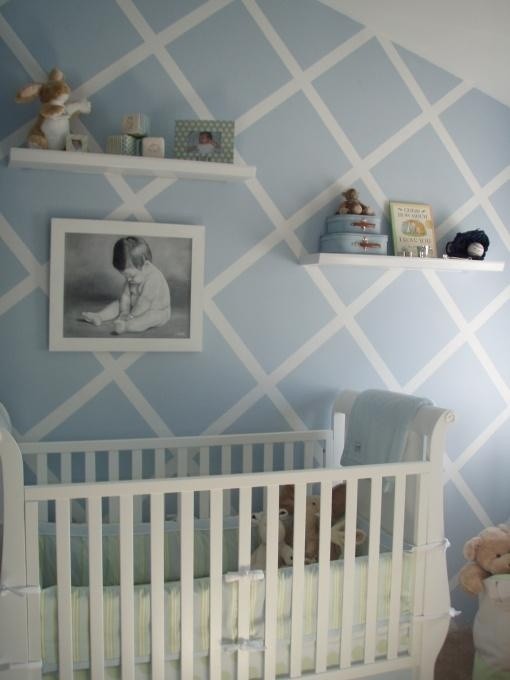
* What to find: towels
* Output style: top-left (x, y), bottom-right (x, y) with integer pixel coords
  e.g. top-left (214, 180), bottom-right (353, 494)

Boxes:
top-left (340, 388), bottom-right (433, 494)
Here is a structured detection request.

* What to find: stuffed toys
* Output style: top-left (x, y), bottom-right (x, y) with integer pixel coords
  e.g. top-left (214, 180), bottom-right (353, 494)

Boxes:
top-left (339, 188), bottom-right (375, 215)
top-left (15, 68), bottom-right (91, 150)
top-left (251, 482), bottom-right (366, 569)
top-left (457, 520), bottom-right (510, 595)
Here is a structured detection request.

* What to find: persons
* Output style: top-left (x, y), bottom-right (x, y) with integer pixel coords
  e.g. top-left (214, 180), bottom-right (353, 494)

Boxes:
top-left (195, 133), bottom-right (216, 151)
top-left (80, 235), bottom-right (171, 335)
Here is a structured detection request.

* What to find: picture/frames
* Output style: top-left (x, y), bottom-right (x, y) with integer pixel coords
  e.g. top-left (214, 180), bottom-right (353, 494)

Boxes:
top-left (389, 200), bottom-right (438, 258)
top-left (171, 117), bottom-right (238, 164)
top-left (49, 217), bottom-right (206, 354)
